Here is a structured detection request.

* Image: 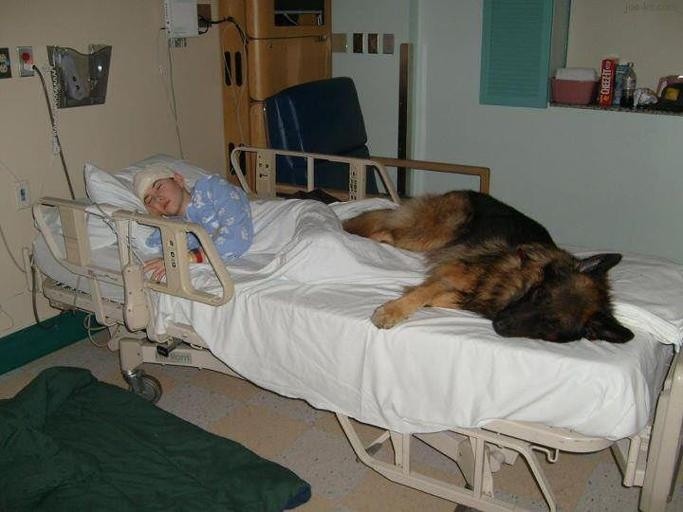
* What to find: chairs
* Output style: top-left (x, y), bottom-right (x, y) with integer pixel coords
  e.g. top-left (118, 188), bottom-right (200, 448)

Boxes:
top-left (264, 76), bottom-right (490, 199)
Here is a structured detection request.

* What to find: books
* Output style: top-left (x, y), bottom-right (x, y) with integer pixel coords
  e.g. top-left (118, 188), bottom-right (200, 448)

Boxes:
top-left (596, 56), bottom-right (616, 107)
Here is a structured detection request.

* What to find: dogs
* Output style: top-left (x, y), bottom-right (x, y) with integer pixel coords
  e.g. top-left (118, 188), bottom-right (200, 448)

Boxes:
top-left (343, 189), bottom-right (635, 343)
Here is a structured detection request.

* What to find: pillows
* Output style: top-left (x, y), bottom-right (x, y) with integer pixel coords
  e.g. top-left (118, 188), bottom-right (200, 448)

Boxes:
top-left (81, 155), bottom-right (212, 265)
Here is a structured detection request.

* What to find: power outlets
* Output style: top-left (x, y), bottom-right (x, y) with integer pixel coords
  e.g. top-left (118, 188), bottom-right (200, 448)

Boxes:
top-left (16, 44), bottom-right (37, 78)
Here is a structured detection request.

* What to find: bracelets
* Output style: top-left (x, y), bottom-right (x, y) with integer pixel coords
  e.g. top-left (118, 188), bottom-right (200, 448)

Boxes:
top-left (188, 247), bottom-right (203, 264)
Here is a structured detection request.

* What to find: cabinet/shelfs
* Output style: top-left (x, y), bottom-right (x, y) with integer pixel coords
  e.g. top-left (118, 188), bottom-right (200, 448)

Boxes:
top-left (481, 0), bottom-right (554, 110)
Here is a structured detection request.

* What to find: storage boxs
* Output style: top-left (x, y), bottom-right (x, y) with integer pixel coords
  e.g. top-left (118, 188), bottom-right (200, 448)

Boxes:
top-left (549, 76), bottom-right (592, 105)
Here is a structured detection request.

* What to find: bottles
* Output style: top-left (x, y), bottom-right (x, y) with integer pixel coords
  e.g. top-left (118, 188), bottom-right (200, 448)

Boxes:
top-left (620, 62), bottom-right (636, 109)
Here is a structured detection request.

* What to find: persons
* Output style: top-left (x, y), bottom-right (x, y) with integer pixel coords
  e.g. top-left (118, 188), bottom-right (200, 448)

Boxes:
top-left (134, 164), bottom-right (253, 285)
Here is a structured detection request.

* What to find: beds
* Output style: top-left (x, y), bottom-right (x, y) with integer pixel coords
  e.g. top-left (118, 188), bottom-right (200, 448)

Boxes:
top-left (33, 147), bottom-right (661, 511)
